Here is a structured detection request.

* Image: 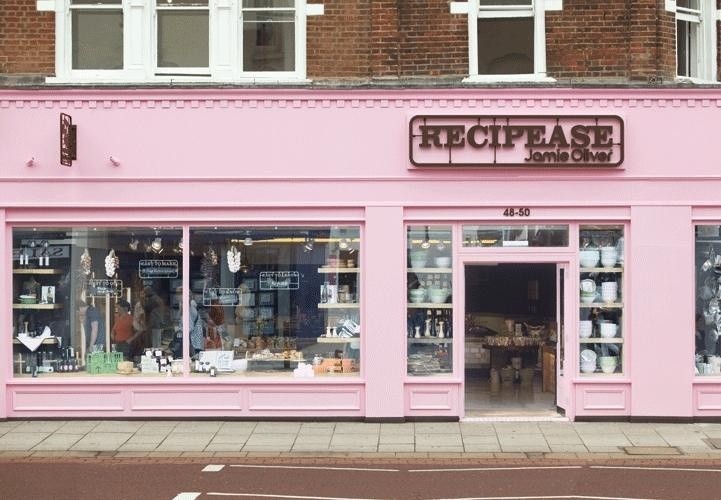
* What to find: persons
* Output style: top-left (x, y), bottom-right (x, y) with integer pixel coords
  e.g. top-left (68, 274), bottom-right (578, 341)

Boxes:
top-left (133, 288), bottom-right (152, 350)
top-left (110, 299), bottom-right (143, 361)
top-left (74, 300), bottom-right (105, 352)
top-left (174, 287), bottom-right (204, 360)
top-left (46, 287), bottom-right (54, 304)
top-left (141, 286), bottom-right (164, 348)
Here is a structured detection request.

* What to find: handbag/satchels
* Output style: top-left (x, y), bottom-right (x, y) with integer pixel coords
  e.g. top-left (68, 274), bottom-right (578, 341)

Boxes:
top-left (169, 330), bottom-right (195, 359)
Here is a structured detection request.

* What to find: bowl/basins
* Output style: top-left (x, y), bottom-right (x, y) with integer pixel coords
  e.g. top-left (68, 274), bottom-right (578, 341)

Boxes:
top-left (409, 249), bottom-right (452, 303)
top-left (579, 246), bottom-right (619, 376)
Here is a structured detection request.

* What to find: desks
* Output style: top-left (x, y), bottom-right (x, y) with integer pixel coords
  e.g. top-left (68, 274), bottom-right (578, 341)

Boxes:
top-left (463, 225), bottom-right (569, 393)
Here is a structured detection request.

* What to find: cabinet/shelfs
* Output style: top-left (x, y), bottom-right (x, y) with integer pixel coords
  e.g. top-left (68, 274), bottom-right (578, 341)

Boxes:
top-left (406, 225), bottom-right (454, 375)
top-left (580, 224), bottom-right (623, 375)
top-left (10, 225), bottom-right (185, 378)
top-left (189, 226), bottom-right (360, 379)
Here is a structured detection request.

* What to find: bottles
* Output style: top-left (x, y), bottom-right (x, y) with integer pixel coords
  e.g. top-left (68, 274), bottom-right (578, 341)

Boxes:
top-left (20, 239), bottom-right (50, 269)
top-left (24, 346), bottom-right (82, 373)
top-left (407, 308), bottom-right (452, 339)
